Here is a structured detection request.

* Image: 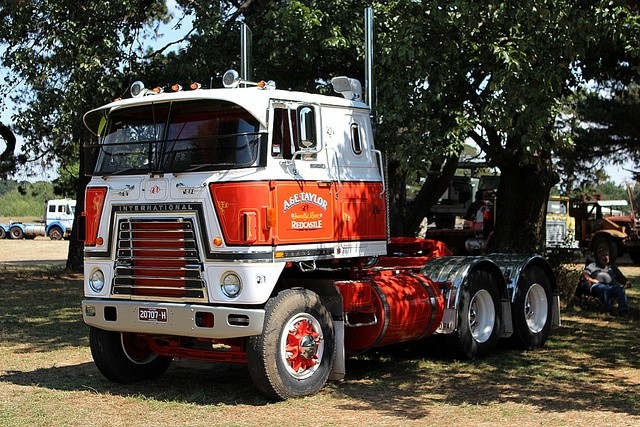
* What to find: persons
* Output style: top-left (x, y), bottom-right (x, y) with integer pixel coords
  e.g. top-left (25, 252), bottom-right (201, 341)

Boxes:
top-left (582, 247), bottom-right (633, 319)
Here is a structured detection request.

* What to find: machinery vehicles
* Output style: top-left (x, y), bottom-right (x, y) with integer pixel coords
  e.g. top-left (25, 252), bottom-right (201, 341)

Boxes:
top-left (427, 162), bottom-right (501, 256)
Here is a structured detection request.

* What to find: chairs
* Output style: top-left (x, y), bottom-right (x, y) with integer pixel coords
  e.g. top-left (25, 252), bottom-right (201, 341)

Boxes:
top-left (580, 258), bottom-right (625, 314)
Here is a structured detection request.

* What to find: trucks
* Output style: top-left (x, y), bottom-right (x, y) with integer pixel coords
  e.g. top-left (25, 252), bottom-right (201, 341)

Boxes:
top-left (546, 195), bottom-right (580, 253)
top-left (0, 197), bottom-right (77, 239)
top-left (569, 194), bottom-right (640, 266)
top-left (81, 7), bottom-right (561, 401)
